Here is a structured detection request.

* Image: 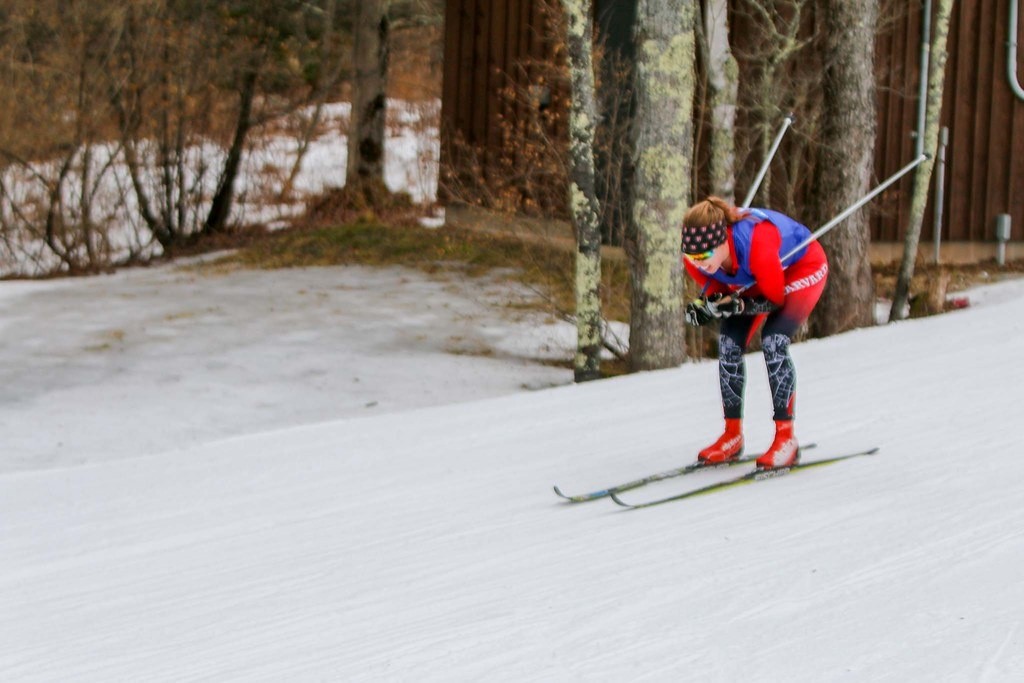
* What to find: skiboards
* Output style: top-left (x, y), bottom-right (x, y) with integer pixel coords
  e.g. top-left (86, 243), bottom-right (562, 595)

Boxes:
top-left (553, 440), bottom-right (881, 509)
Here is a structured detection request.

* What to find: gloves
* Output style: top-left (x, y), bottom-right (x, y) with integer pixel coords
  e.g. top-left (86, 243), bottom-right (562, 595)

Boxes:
top-left (684, 302), bottom-right (711, 327)
top-left (705, 292), bottom-right (745, 318)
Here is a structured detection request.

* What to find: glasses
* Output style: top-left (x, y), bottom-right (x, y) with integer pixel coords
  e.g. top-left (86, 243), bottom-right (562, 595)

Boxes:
top-left (680, 231), bottom-right (725, 262)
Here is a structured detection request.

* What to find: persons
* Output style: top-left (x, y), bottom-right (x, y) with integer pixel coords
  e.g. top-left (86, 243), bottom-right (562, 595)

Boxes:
top-left (681, 194), bottom-right (829, 469)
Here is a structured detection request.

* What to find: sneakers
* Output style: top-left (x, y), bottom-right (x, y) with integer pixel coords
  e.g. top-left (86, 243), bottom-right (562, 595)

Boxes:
top-left (699, 417), bottom-right (744, 465)
top-left (756, 418), bottom-right (800, 469)
